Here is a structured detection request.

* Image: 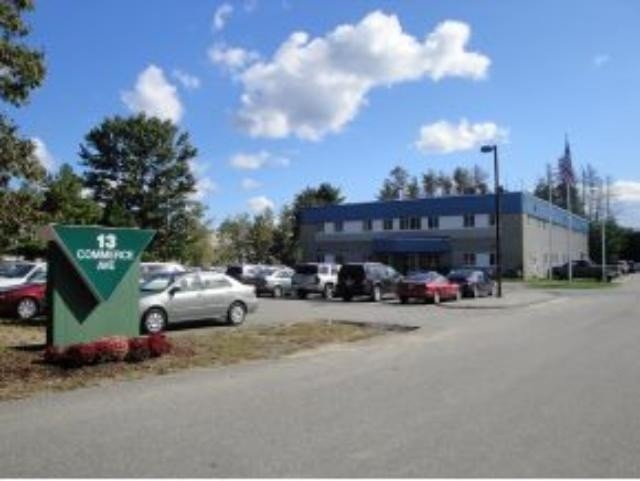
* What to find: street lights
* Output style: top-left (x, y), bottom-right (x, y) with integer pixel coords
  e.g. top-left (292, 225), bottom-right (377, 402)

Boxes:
top-left (479, 142), bottom-right (502, 299)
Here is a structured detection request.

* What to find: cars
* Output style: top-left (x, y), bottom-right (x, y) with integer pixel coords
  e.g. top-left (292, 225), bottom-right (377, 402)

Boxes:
top-left (446, 266), bottom-right (499, 299)
top-left (0, 260), bottom-right (295, 335)
top-left (395, 271), bottom-right (463, 304)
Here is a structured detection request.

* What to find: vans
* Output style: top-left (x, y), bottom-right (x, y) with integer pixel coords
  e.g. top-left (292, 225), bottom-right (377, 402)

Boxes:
top-left (336, 261), bottom-right (403, 302)
top-left (291, 261), bottom-right (344, 301)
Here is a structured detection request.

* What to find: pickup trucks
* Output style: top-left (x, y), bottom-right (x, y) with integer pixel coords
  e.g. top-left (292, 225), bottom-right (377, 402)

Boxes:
top-left (550, 259), bottom-right (632, 282)
